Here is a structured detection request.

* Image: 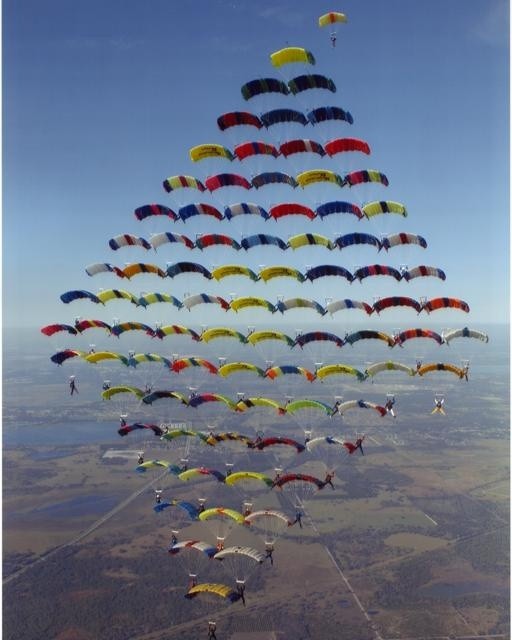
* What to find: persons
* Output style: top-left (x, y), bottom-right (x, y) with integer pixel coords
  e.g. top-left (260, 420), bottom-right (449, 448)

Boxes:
top-left (331, 32), bottom-right (337, 47)
top-left (207, 624), bottom-right (217, 640)
